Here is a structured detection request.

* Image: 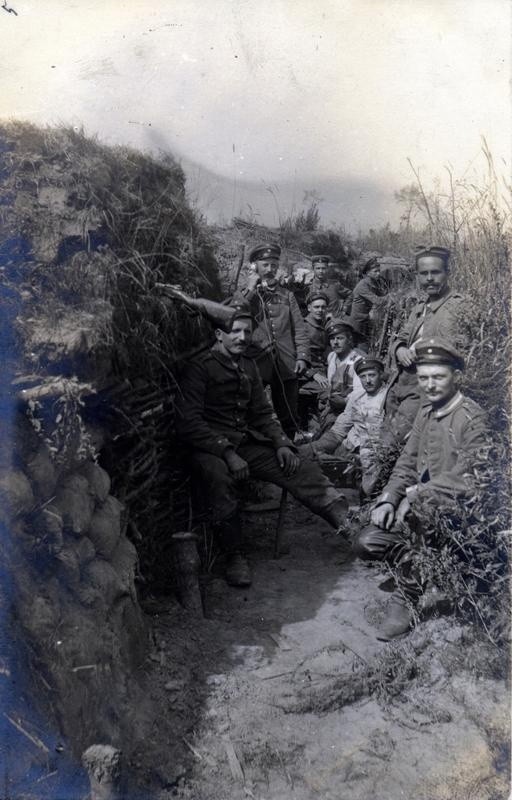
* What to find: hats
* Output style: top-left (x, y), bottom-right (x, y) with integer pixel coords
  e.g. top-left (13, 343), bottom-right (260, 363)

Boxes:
top-left (311, 255), bottom-right (329, 267)
top-left (249, 243), bottom-right (281, 265)
top-left (414, 245), bottom-right (451, 262)
top-left (353, 356), bottom-right (384, 375)
top-left (305, 291), bottom-right (329, 306)
top-left (324, 318), bottom-right (354, 337)
top-left (413, 336), bottom-right (465, 371)
top-left (362, 256), bottom-right (380, 274)
top-left (209, 290), bottom-right (254, 332)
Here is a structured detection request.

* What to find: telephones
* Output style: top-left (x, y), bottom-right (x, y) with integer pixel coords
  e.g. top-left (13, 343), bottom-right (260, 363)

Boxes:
top-left (247, 262), bottom-right (268, 292)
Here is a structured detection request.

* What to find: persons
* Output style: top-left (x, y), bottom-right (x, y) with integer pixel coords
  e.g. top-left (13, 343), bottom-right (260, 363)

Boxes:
top-left (298, 291), bottom-right (332, 418)
top-left (178, 302), bottom-right (359, 587)
top-left (228, 242), bottom-right (310, 437)
top-left (353, 361), bottom-right (489, 561)
top-left (360, 255), bottom-right (474, 497)
top-left (322, 316), bottom-right (366, 402)
top-left (303, 359), bottom-right (384, 463)
top-left (352, 257), bottom-right (388, 317)
top-left (307, 256), bottom-right (353, 317)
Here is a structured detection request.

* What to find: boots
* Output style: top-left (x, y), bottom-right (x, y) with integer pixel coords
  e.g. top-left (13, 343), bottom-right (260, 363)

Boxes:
top-left (207, 524), bottom-right (252, 586)
top-left (321, 499), bottom-right (365, 545)
top-left (375, 550), bottom-right (429, 642)
